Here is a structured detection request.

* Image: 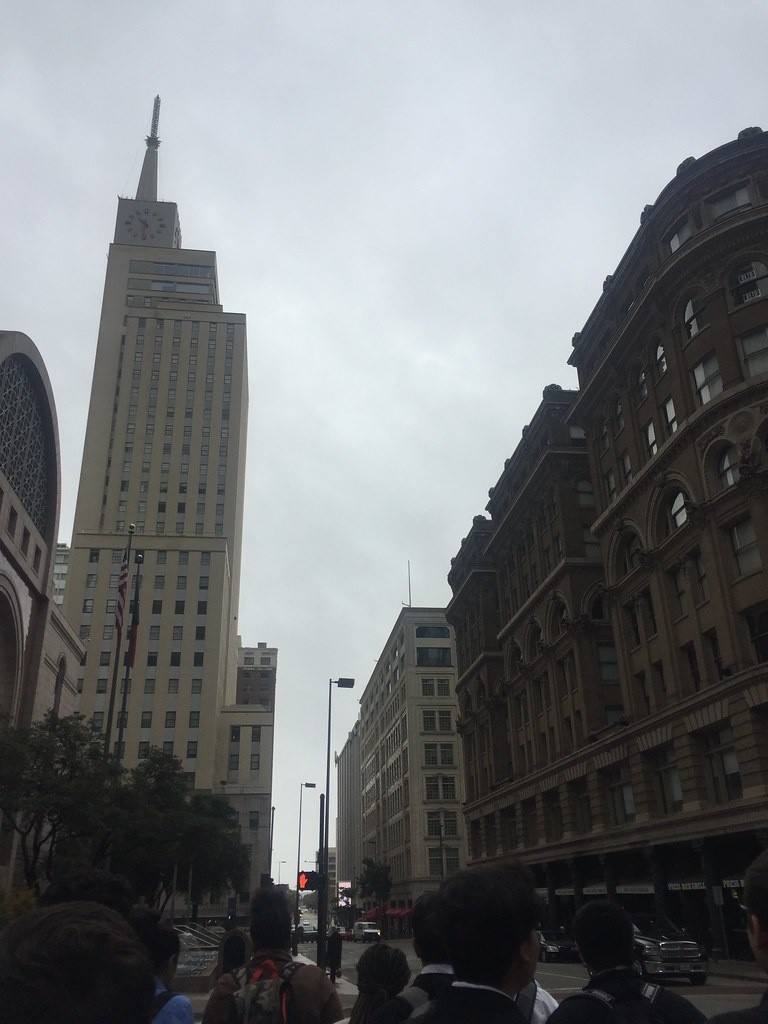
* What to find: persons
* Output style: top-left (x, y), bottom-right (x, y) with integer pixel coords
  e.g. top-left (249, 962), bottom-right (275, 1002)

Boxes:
top-left (0, 866), bottom-right (348, 1024)
top-left (334, 943), bottom-right (411, 1024)
top-left (546, 898), bottom-right (708, 1024)
top-left (368, 890), bottom-right (461, 1024)
top-left (399, 858), bottom-right (560, 1024)
top-left (708, 849), bottom-right (768, 1024)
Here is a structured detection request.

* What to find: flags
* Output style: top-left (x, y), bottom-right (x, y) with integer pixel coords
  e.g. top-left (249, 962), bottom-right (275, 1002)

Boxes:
top-left (128, 583), bottom-right (139, 669)
top-left (115, 544), bottom-right (128, 638)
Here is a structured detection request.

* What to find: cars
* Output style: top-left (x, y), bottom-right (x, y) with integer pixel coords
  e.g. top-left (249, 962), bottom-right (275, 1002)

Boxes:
top-left (291, 921), bottom-right (380, 943)
top-left (536, 912), bottom-right (707, 985)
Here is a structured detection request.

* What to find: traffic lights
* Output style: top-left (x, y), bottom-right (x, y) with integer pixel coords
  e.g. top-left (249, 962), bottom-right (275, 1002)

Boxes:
top-left (299, 871), bottom-right (315, 890)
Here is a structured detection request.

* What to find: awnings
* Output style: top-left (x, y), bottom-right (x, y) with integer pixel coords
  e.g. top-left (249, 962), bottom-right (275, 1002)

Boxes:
top-left (555, 870), bottom-right (748, 896)
top-left (356, 901), bottom-right (412, 922)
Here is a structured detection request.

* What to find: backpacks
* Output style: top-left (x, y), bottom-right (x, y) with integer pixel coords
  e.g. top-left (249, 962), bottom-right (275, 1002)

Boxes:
top-left (230, 962), bottom-right (303, 1024)
top-left (560, 981), bottom-right (666, 1024)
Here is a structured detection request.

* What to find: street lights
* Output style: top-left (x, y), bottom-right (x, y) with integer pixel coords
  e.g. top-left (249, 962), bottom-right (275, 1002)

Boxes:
top-left (324, 678), bottom-right (354, 972)
top-left (278, 861), bottom-right (286, 884)
top-left (295, 782), bottom-right (316, 929)
